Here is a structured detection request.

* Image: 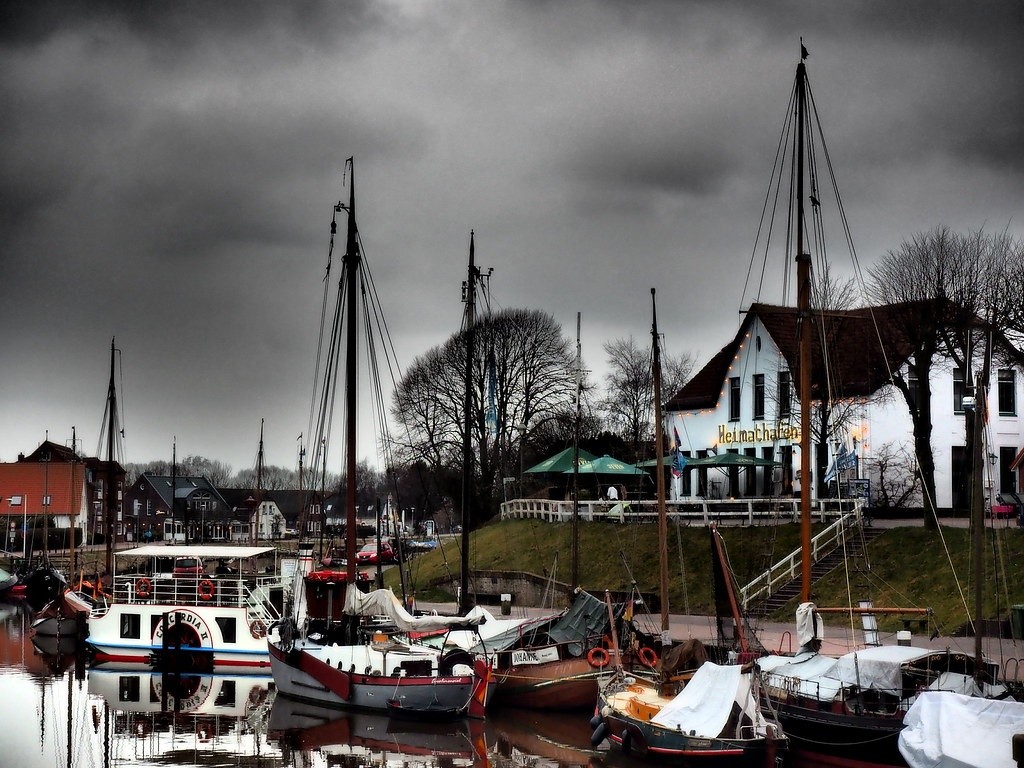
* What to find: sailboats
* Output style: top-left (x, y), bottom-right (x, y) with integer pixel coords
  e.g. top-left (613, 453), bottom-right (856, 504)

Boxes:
top-left (0, 34), bottom-right (1024, 764)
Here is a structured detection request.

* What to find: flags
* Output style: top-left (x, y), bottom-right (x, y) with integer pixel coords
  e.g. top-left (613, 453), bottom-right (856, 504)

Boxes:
top-left (821, 435), bottom-right (856, 482)
top-left (674, 426), bottom-right (684, 478)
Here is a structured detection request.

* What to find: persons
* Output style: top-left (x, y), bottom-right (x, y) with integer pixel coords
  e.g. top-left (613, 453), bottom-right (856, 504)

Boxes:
top-left (793, 470), bottom-right (802, 522)
top-left (605, 483), bottom-right (619, 510)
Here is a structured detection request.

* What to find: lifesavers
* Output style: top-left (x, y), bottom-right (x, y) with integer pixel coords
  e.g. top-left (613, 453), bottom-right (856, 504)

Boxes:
top-left (198, 581), bottom-right (215, 600)
top-left (637, 647), bottom-right (658, 668)
top-left (250, 620), bottom-right (267, 638)
top-left (135, 578), bottom-right (152, 598)
top-left (586, 647), bottom-right (610, 668)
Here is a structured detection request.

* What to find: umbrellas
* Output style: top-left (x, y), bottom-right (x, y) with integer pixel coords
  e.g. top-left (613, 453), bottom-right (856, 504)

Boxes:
top-left (629, 453), bottom-right (699, 500)
top-left (560, 453), bottom-right (651, 502)
top-left (682, 450), bottom-right (783, 500)
top-left (524, 447), bottom-right (598, 497)
